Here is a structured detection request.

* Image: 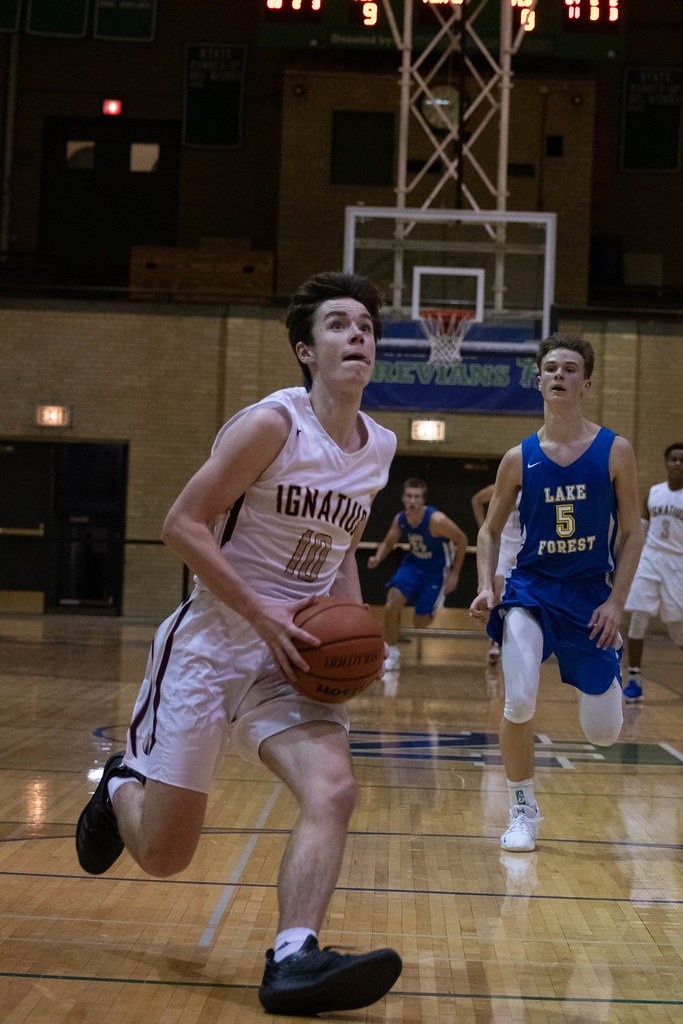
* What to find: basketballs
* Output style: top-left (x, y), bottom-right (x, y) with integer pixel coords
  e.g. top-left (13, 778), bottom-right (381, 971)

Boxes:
top-left (275, 594), bottom-right (385, 705)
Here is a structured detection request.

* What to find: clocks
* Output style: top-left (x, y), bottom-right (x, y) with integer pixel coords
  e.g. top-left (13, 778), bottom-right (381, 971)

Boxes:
top-left (419, 84), bottom-right (471, 134)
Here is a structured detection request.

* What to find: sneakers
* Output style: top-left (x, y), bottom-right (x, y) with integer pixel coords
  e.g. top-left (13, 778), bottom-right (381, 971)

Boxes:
top-left (259, 935), bottom-right (403, 1017)
top-left (498, 798), bottom-right (545, 852)
top-left (383, 646), bottom-right (403, 670)
top-left (485, 641), bottom-right (500, 666)
top-left (623, 678), bottom-right (643, 702)
top-left (75, 751), bottom-right (147, 875)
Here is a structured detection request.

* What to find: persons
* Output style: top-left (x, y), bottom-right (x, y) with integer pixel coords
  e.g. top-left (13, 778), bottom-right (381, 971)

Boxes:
top-left (624, 442), bottom-right (683, 701)
top-left (467, 333), bottom-right (644, 851)
top-left (472, 485), bottom-right (523, 663)
top-left (368, 478), bottom-right (467, 670)
top-left (76, 272), bottom-right (403, 1016)
